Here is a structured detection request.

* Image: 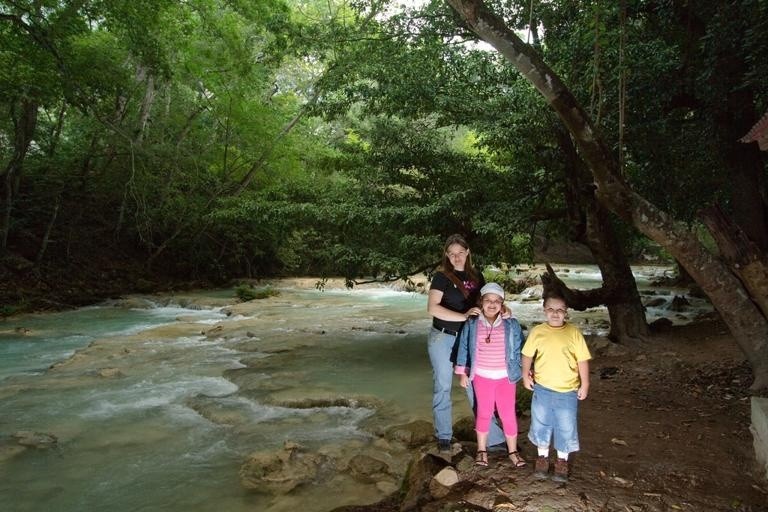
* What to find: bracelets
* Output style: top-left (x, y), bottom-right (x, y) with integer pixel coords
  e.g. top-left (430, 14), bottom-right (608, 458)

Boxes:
top-left (464, 313), bottom-right (469, 320)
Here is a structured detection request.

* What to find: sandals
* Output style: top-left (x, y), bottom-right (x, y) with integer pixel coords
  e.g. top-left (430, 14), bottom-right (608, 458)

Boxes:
top-left (474, 449), bottom-right (490, 468)
top-left (507, 450), bottom-right (529, 469)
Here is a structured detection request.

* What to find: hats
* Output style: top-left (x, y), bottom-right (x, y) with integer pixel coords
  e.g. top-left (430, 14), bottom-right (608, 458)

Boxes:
top-left (479, 282), bottom-right (505, 300)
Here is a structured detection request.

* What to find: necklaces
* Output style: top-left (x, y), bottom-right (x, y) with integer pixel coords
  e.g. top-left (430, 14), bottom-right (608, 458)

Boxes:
top-left (452, 269), bottom-right (468, 285)
top-left (484, 315), bottom-right (493, 344)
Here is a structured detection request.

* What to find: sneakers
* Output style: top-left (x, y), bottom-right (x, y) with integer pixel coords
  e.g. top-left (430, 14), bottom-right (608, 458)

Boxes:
top-left (489, 440), bottom-right (522, 452)
top-left (534, 455), bottom-right (550, 478)
top-left (439, 439), bottom-right (451, 454)
top-left (551, 458), bottom-right (570, 483)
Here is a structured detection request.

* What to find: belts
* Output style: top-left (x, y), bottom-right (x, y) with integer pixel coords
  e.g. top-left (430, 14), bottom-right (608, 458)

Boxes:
top-left (433, 324), bottom-right (456, 337)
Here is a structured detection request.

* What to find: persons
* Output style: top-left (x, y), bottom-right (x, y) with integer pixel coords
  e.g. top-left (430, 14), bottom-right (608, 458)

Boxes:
top-left (453, 283), bottom-right (533, 469)
top-left (520, 293), bottom-right (593, 483)
top-left (426, 233), bottom-right (521, 454)
